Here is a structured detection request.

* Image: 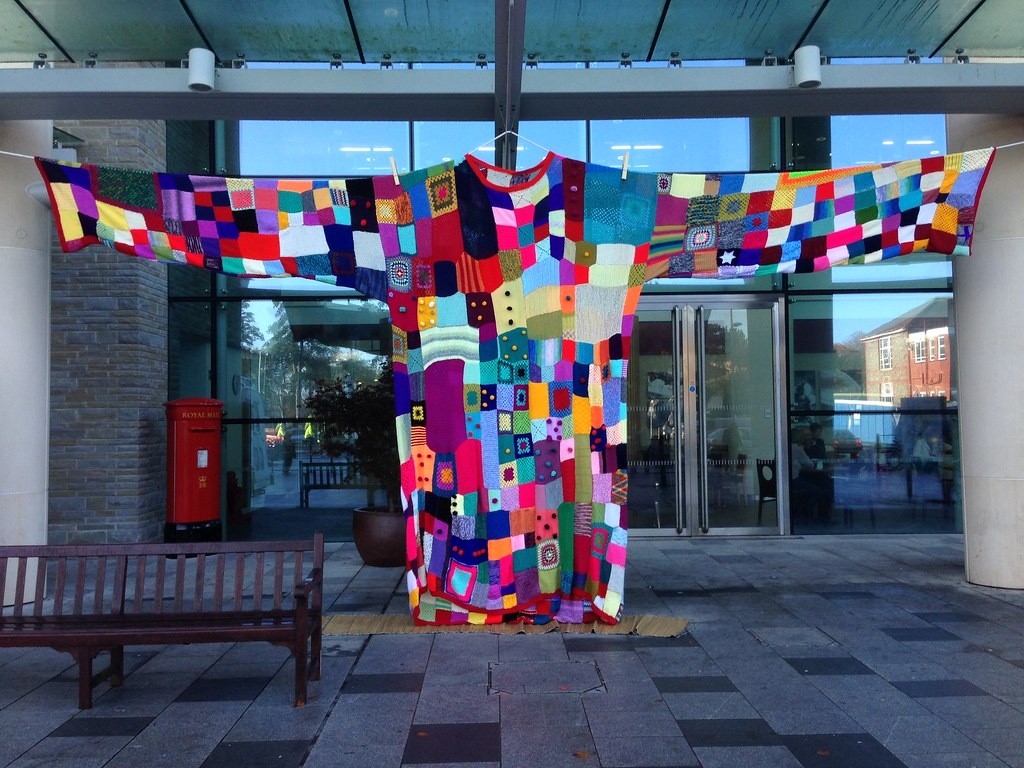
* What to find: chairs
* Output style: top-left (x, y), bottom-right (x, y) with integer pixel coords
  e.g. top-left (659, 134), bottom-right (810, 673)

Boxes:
top-left (834, 457), bottom-right (873, 531)
top-left (754, 459), bottom-right (779, 525)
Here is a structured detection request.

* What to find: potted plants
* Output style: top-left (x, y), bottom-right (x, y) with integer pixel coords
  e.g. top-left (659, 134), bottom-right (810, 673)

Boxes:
top-left (305, 357), bottom-right (408, 567)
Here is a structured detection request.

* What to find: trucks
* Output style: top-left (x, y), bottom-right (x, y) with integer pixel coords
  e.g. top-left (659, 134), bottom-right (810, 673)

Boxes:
top-left (219, 387), bottom-right (274, 508)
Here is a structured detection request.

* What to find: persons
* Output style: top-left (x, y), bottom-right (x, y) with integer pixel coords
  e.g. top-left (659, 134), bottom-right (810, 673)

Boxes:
top-left (282, 431), bottom-right (297, 475)
top-left (790, 422), bottom-right (835, 525)
top-left (913, 425), bottom-right (953, 457)
top-left (723, 422), bottom-right (744, 470)
top-left (276, 422), bottom-right (285, 437)
top-left (663, 411), bottom-right (675, 445)
top-left (304, 414), bottom-right (316, 448)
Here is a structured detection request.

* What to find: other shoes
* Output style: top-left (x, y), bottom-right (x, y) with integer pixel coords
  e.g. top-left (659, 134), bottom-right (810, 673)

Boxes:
top-left (284, 471), bottom-right (291, 476)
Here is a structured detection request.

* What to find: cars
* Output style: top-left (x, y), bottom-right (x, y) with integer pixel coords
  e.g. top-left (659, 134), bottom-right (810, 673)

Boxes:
top-left (832, 429), bottom-right (864, 461)
top-left (261, 426), bottom-right (331, 454)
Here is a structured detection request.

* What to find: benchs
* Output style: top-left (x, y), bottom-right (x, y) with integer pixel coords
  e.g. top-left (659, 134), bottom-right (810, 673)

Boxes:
top-left (298, 459), bottom-right (377, 508)
top-left (0, 529), bottom-right (323, 710)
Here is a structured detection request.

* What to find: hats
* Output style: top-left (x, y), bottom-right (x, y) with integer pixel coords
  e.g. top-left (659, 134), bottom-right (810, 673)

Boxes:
top-left (921, 426), bottom-right (942, 437)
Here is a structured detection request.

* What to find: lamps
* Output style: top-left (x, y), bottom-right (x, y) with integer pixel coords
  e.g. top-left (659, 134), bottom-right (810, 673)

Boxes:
top-left (794, 45), bottom-right (821, 88)
top-left (186, 47), bottom-right (215, 91)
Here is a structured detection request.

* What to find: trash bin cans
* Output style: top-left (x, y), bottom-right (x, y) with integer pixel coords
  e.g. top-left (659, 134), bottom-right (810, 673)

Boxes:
top-left (798, 463), bottom-right (835, 520)
